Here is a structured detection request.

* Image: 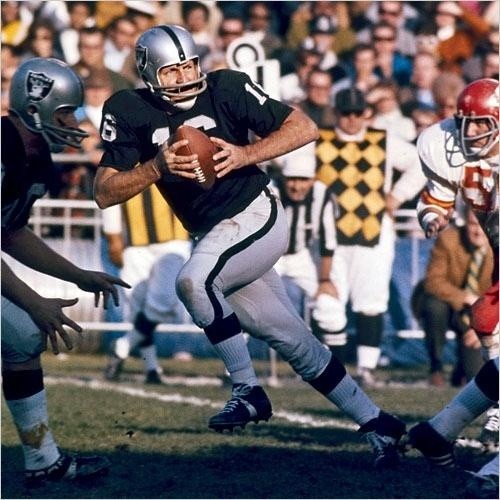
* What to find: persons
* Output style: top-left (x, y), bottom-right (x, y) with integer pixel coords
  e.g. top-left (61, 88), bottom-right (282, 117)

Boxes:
top-left (0, 57), bottom-right (131, 488)
top-left (0, 0), bottom-right (498, 387)
top-left (412, 78), bottom-right (499, 499)
top-left (91, 25), bottom-right (409, 471)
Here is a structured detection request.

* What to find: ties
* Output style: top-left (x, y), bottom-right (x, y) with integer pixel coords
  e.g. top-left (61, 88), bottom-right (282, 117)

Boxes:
top-left (459, 250), bottom-right (481, 326)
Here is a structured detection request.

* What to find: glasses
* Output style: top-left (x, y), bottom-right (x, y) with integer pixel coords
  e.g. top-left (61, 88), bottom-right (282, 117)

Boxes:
top-left (341, 112), bottom-right (365, 118)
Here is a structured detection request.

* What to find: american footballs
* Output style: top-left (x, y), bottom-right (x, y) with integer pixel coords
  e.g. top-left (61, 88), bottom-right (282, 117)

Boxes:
top-left (173, 125), bottom-right (218, 190)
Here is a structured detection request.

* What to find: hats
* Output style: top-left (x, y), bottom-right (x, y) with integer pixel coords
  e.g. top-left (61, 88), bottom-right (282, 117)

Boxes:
top-left (335, 87), bottom-right (364, 113)
top-left (307, 13), bottom-right (338, 35)
top-left (282, 152), bottom-right (317, 179)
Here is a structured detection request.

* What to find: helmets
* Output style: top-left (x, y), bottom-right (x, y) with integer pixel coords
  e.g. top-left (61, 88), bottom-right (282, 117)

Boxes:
top-left (135, 25), bottom-right (208, 103)
top-left (8, 58), bottom-right (90, 150)
top-left (454, 77), bottom-right (500, 160)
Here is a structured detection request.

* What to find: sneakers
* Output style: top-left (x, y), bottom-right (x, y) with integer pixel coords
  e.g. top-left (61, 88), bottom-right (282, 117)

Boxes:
top-left (208, 382), bottom-right (272, 432)
top-left (479, 405), bottom-right (499, 445)
top-left (23, 444), bottom-right (112, 494)
top-left (104, 339), bottom-right (124, 381)
top-left (358, 408), bottom-right (458, 472)
